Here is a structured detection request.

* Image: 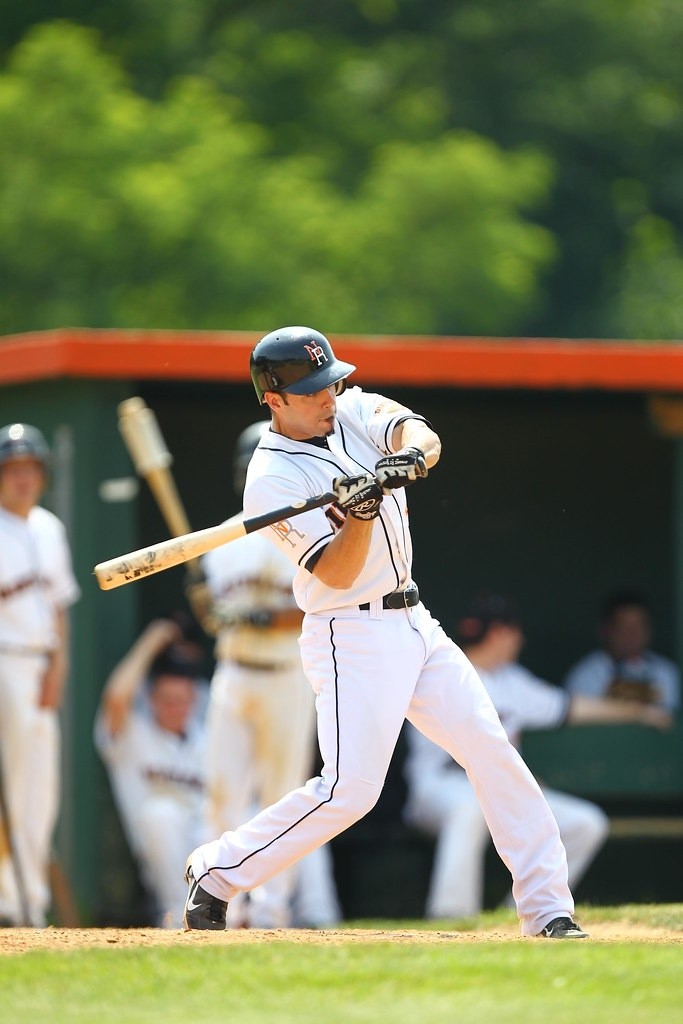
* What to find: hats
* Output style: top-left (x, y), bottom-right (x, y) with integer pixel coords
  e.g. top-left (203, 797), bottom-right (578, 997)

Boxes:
top-left (0, 422), bottom-right (51, 468)
top-left (250, 326), bottom-right (356, 406)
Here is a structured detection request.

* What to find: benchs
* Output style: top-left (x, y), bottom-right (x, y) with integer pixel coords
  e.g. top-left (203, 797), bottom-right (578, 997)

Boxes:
top-left (519, 716), bottom-right (683, 837)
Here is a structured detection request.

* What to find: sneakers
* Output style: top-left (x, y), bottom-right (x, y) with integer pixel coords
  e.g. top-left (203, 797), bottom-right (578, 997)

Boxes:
top-left (540, 917), bottom-right (589, 939)
top-left (184, 851), bottom-right (228, 930)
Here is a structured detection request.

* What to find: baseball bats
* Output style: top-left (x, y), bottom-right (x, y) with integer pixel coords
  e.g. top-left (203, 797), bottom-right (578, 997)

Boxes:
top-left (93, 474), bottom-right (376, 593)
top-left (119, 394), bottom-right (222, 631)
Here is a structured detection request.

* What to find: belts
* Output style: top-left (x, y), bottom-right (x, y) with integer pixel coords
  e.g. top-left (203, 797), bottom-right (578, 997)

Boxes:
top-left (359, 580), bottom-right (419, 610)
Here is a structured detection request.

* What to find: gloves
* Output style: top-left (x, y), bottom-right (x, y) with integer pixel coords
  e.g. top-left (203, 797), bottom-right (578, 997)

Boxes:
top-left (375, 447), bottom-right (428, 490)
top-left (333, 473), bottom-right (383, 521)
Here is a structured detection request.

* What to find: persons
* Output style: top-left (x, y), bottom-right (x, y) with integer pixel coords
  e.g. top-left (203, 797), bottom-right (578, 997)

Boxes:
top-left (1, 422), bottom-right (81, 927)
top-left (92, 419), bottom-right (683, 929)
top-left (184, 325), bottom-right (589, 939)
